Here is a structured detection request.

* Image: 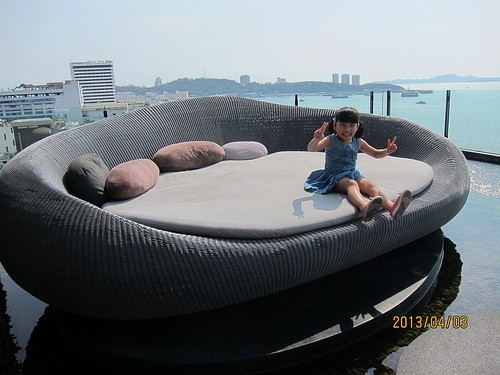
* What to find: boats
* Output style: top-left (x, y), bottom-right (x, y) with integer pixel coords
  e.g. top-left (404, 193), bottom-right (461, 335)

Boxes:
top-left (416, 100), bottom-right (426, 104)
top-left (400, 90), bottom-right (420, 97)
top-left (332, 94), bottom-right (349, 99)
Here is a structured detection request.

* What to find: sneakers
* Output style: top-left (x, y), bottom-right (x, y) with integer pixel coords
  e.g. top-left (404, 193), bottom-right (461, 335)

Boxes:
top-left (389, 190), bottom-right (412, 218)
top-left (360, 196), bottom-right (383, 222)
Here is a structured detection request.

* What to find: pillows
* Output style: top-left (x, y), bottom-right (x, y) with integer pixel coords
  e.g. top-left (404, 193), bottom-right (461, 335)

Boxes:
top-left (68, 152), bottom-right (111, 204)
top-left (221, 141), bottom-right (268, 160)
top-left (105, 158), bottom-right (160, 200)
top-left (153, 141), bottom-right (226, 172)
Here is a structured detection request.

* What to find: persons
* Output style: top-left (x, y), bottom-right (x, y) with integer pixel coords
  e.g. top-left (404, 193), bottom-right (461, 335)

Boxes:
top-left (304, 107), bottom-right (412, 222)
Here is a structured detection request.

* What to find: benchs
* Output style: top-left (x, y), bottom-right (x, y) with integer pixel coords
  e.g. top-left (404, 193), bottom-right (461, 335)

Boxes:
top-left (0, 95), bottom-right (469, 324)
